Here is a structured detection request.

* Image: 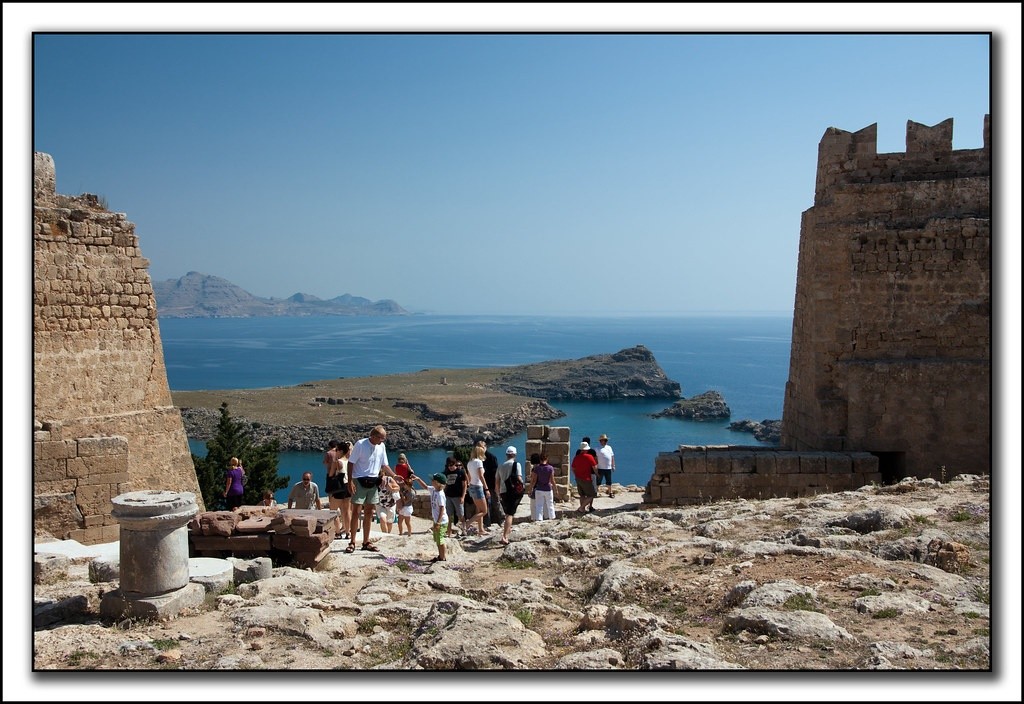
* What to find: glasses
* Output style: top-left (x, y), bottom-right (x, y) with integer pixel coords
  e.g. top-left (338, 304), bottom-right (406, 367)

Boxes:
top-left (446, 463), bottom-right (455, 467)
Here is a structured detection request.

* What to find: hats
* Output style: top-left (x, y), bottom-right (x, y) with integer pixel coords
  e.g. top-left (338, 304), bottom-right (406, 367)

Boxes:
top-left (599, 434), bottom-right (610, 441)
top-left (428, 472), bottom-right (448, 484)
top-left (506, 445), bottom-right (517, 455)
top-left (579, 441), bottom-right (590, 451)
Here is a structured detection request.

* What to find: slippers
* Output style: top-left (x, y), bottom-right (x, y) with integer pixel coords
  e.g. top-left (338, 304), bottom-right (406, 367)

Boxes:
top-left (479, 531), bottom-right (490, 535)
top-left (576, 508), bottom-right (589, 516)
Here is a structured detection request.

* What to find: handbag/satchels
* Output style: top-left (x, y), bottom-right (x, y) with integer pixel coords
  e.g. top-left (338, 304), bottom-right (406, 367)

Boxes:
top-left (324, 459), bottom-right (345, 494)
top-left (357, 476), bottom-right (378, 488)
top-left (388, 476), bottom-right (401, 500)
top-left (239, 467), bottom-right (249, 486)
top-left (504, 461), bottom-right (525, 499)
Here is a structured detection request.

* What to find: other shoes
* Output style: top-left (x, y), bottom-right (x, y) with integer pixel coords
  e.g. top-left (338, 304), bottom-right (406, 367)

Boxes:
top-left (345, 533), bottom-right (351, 539)
top-left (361, 542), bottom-right (380, 552)
top-left (334, 533), bottom-right (342, 539)
top-left (589, 506), bottom-right (595, 511)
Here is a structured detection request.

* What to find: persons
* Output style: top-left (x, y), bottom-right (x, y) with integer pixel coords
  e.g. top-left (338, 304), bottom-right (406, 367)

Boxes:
top-left (236, 457), bottom-right (244, 476)
top-left (256, 490), bottom-right (277, 507)
top-left (409, 472), bottom-right (449, 562)
top-left (571, 441), bottom-right (599, 515)
top-left (344, 426), bottom-right (405, 553)
top-left (322, 439), bottom-right (414, 540)
top-left (594, 433), bottom-right (615, 498)
top-left (575, 437), bottom-right (598, 511)
top-left (442, 439), bottom-right (559, 545)
top-left (223, 456), bottom-right (244, 511)
top-left (287, 471), bottom-right (321, 510)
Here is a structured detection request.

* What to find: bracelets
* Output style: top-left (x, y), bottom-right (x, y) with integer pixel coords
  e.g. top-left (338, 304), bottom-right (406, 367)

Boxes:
top-left (612, 464), bottom-right (615, 467)
top-left (224, 490), bottom-right (228, 494)
top-left (436, 521), bottom-right (440, 525)
top-left (392, 474), bottom-right (396, 478)
top-left (484, 489), bottom-right (489, 491)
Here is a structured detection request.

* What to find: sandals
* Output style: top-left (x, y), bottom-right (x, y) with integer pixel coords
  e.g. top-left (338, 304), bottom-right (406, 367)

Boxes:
top-left (345, 542), bottom-right (355, 552)
top-left (609, 492), bottom-right (615, 498)
